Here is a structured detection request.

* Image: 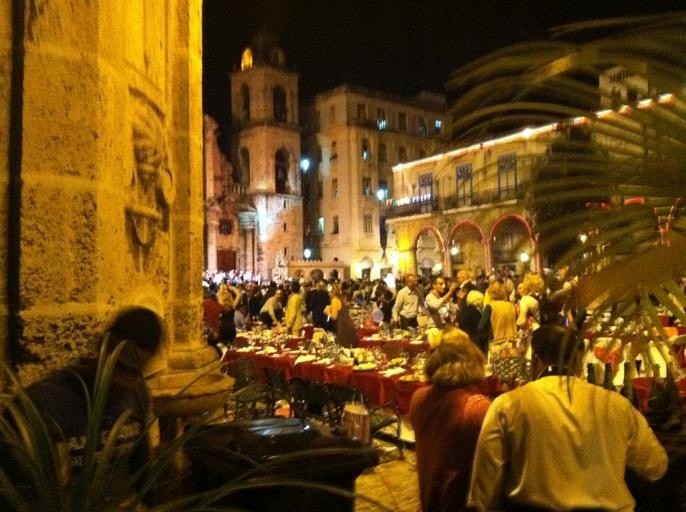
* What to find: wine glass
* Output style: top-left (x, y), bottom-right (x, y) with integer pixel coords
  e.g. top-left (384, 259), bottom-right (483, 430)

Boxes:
top-left (248, 336), bottom-right (305, 352)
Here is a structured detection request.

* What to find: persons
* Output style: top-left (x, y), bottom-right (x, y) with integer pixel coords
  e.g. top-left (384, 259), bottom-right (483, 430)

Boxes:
top-left (468, 325), bottom-right (668, 510)
top-left (1, 307), bottom-right (162, 510)
top-left (203, 263), bottom-right (587, 360)
top-left (408, 328), bottom-right (494, 512)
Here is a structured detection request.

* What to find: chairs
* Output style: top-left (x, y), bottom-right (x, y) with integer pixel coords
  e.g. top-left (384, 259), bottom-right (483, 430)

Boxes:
top-left (226, 318), bottom-right (430, 467)
top-left (492, 355), bottom-right (535, 384)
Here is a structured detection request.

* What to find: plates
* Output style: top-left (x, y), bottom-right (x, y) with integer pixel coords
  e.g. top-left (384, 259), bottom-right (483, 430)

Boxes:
top-left (354, 357), bottom-right (427, 384)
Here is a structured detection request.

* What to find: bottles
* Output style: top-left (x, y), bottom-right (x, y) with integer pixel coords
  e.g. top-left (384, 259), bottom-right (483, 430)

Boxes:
top-left (584, 359), bottom-right (680, 426)
